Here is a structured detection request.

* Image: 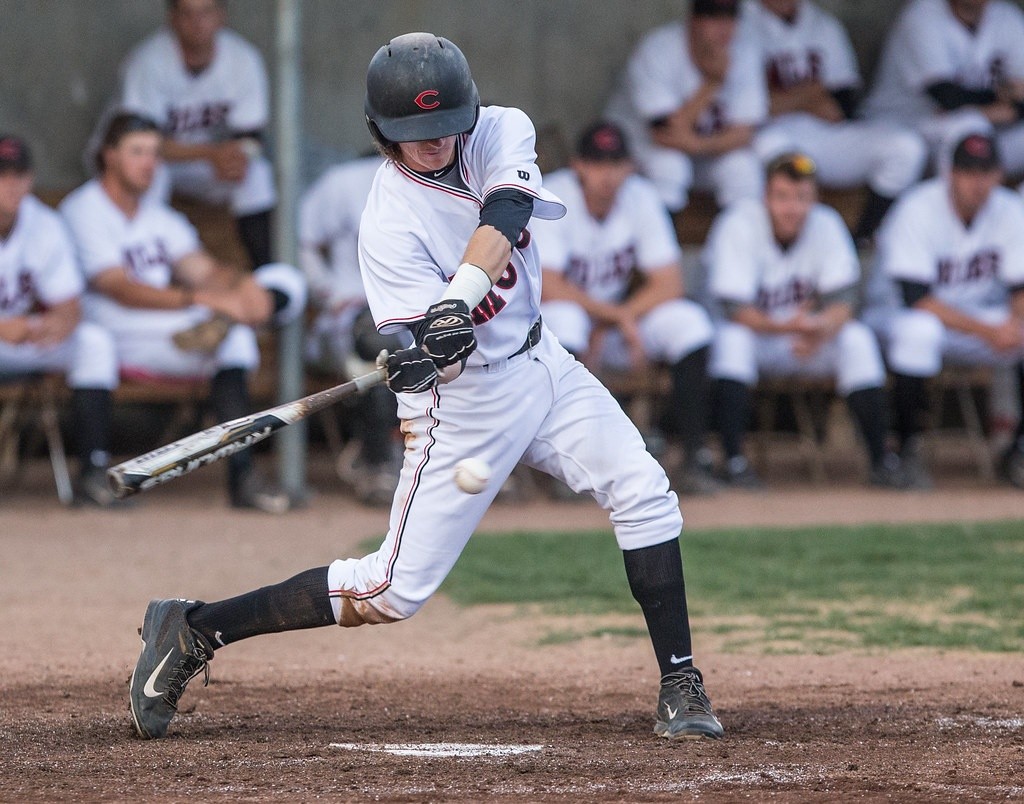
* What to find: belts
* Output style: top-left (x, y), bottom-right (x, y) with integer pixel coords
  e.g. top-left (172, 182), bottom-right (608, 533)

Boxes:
top-left (482, 314), bottom-right (543, 368)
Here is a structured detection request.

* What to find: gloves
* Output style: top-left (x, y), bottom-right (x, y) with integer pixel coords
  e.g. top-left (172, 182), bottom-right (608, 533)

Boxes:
top-left (377, 346), bottom-right (445, 394)
top-left (422, 299), bottom-right (477, 368)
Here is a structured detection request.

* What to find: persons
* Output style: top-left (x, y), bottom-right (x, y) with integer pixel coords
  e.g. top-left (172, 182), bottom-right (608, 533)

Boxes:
top-left (0, 136), bottom-right (122, 505)
top-left (58, 108), bottom-right (307, 514)
top-left (128, 30), bottom-right (724, 747)
top-left (294, 0), bottom-right (1024, 501)
top-left (116, 1), bottom-right (277, 274)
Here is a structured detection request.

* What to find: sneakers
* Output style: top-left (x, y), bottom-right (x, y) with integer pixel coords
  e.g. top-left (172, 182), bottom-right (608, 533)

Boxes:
top-left (127, 599), bottom-right (216, 740)
top-left (652, 666), bottom-right (724, 744)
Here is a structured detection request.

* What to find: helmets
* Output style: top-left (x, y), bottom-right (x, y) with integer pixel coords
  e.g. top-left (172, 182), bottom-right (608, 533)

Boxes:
top-left (364, 33), bottom-right (480, 159)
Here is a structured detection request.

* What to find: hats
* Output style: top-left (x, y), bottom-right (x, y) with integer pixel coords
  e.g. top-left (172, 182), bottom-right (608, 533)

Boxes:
top-left (954, 137), bottom-right (998, 172)
top-left (769, 150), bottom-right (819, 177)
top-left (693, 0), bottom-right (737, 18)
top-left (580, 121), bottom-right (628, 161)
top-left (0, 137), bottom-right (32, 172)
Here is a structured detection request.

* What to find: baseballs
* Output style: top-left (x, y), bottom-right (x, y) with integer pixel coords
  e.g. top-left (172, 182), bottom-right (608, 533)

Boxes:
top-left (453, 456), bottom-right (492, 495)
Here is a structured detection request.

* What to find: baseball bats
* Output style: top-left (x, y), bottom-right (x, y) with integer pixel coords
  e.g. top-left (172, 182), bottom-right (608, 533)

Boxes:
top-left (105, 367), bottom-right (386, 501)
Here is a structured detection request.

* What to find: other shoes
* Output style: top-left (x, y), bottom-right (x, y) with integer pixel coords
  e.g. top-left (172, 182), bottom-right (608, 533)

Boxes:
top-left (662, 444), bottom-right (727, 495)
top-left (76, 464), bottom-right (127, 510)
top-left (719, 452), bottom-right (767, 491)
top-left (869, 448), bottom-right (933, 488)
top-left (352, 451), bottom-right (401, 506)
top-left (1001, 446), bottom-right (1024, 485)
top-left (231, 457), bottom-right (298, 515)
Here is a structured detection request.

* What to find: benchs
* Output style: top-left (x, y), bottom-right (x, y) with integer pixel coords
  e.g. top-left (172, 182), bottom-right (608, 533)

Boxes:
top-left (1, 189), bottom-right (1024, 497)
